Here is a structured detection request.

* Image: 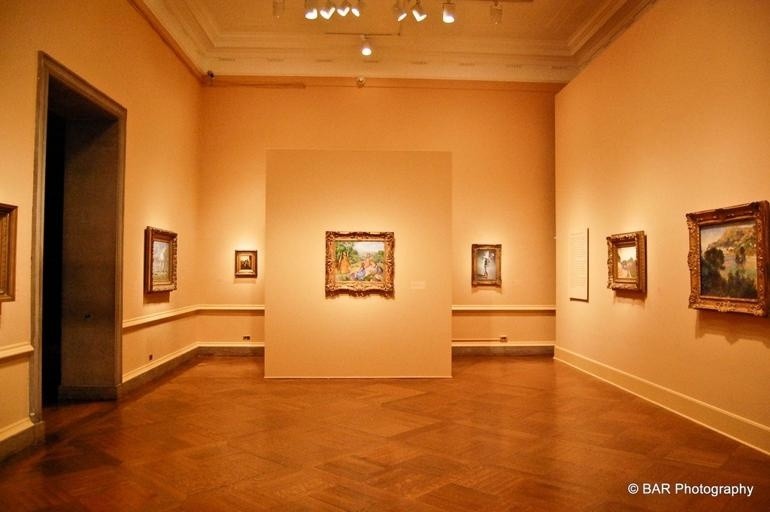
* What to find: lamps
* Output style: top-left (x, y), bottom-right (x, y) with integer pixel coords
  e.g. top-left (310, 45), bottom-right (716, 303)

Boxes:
top-left (391, 0), bottom-right (407, 21)
top-left (490, 0), bottom-right (503, 25)
top-left (337, 0), bottom-right (352, 16)
top-left (320, 0), bottom-right (336, 20)
top-left (359, 33), bottom-right (372, 56)
top-left (410, 0), bottom-right (427, 23)
top-left (351, 0), bottom-right (367, 16)
top-left (303, 0), bottom-right (317, 20)
top-left (441, 0), bottom-right (456, 23)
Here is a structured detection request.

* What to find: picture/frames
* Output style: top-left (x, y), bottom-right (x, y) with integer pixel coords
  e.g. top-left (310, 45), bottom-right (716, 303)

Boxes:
top-left (472, 244), bottom-right (501, 286)
top-left (235, 250), bottom-right (257, 277)
top-left (685, 200), bottom-right (770, 318)
top-left (325, 231), bottom-right (394, 295)
top-left (0, 202), bottom-right (18, 302)
top-left (145, 226), bottom-right (177, 293)
top-left (606, 231), bottom-right (646, 294)
